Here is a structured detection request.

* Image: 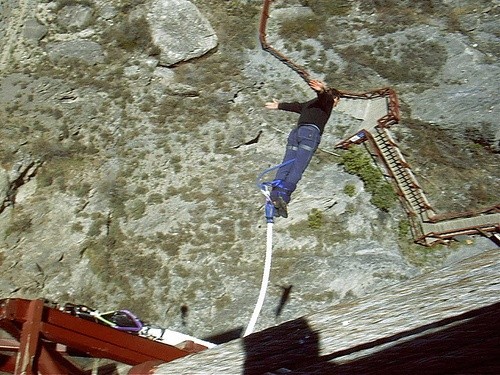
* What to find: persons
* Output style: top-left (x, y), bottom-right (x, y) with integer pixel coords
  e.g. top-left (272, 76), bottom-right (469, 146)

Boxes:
top-left (263, 79), bottom-right (341, 220)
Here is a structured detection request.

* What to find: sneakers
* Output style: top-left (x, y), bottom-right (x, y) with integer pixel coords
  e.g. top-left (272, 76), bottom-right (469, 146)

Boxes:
top-left (274, 196), bottom-right (289, 218)
